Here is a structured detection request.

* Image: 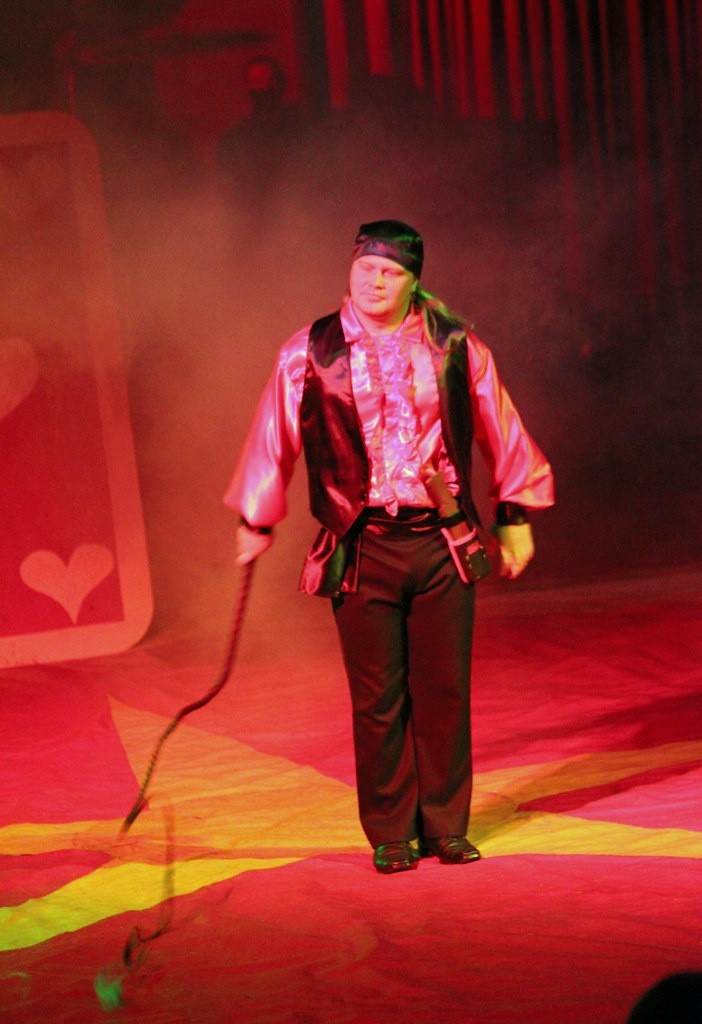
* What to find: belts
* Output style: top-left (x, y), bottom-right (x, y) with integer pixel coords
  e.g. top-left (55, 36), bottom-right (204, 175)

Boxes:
top-left (362, 513), bottom-right (439, 540)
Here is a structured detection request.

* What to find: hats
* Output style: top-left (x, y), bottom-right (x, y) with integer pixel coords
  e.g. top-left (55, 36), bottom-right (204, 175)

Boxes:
top-left (352, 219), bottom-right (424, 277)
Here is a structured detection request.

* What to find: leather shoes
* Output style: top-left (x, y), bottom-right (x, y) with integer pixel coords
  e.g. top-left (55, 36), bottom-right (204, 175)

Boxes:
top-left (373, 840), bottom-right (413, 874)
top-left (419, 836), bottom-right (480, 864)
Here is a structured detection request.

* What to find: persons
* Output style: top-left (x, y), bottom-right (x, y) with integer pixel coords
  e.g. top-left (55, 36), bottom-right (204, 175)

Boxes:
top-left (627, 969), bottom-right (702, 1024)
top-left (224, 218), bottom-right (555, 875)
